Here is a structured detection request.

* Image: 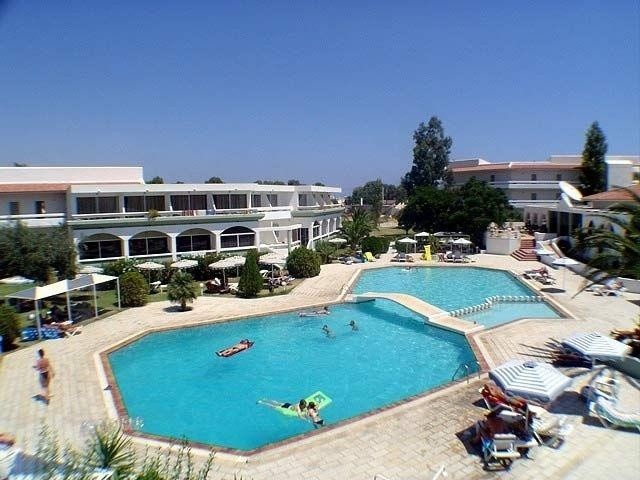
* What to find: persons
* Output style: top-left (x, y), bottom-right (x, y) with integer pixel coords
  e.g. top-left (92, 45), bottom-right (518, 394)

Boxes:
top-left (37, 202), bottom-right (46, 218)
top-left (343, 320), bottom-right (359, 331)
top-left (306, 402), bottom-right (326, 428)
top-left (299, 306), bottom-right (330, 317)
top-left (321, 324), bottom-right (336, 341)
top-left (261, 267), bottom-right (285, 281)
top-left (599, 279), bottom-right (624, 290)
top-left (217, 338), bottom-right (249, 356)
top-left (32, 349), bottom-right (56, 400)
top-left (534, 267), bottom-right (547, 277)
top-left (472, 382), bottom-right (533, 445)
top-left (488, 358), bottom-right (573, 430)
top-left (256, 395), bottom-right (309, 423)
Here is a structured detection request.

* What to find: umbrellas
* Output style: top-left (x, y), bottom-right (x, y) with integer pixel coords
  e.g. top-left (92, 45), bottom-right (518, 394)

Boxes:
top-left (447, 236), bottom-right (473, 253)
top-left (397, 235), bottom-right (418, 253)
top-left (225, 255), bottom-right (246, 277)
top-left (208, 260), bottom-right (236, 282)
top-left (552, 256), bottom-right (580, 288)
top-left (561, 328), bottom-right (632, 405)
top-left (135, 261), bottom-right (166, 294)
top-left (77, 265), bottom-right (104, 274)
top-left (328, 237), bottom-right (347, 258)
top-left (258, 254), bottom-right (286, 277)
top-left (169, 258), bottom-right (199, 272)
top-left (0, 275), bottom-right (35, 284)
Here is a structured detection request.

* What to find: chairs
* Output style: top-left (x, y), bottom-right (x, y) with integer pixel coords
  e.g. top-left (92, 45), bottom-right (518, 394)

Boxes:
top-left (472, 380), bottom-right (570, 470)
top-left (588, 279), bottom-right (627, 296)
top-left (584, 363), bottom-right (640, 431)
